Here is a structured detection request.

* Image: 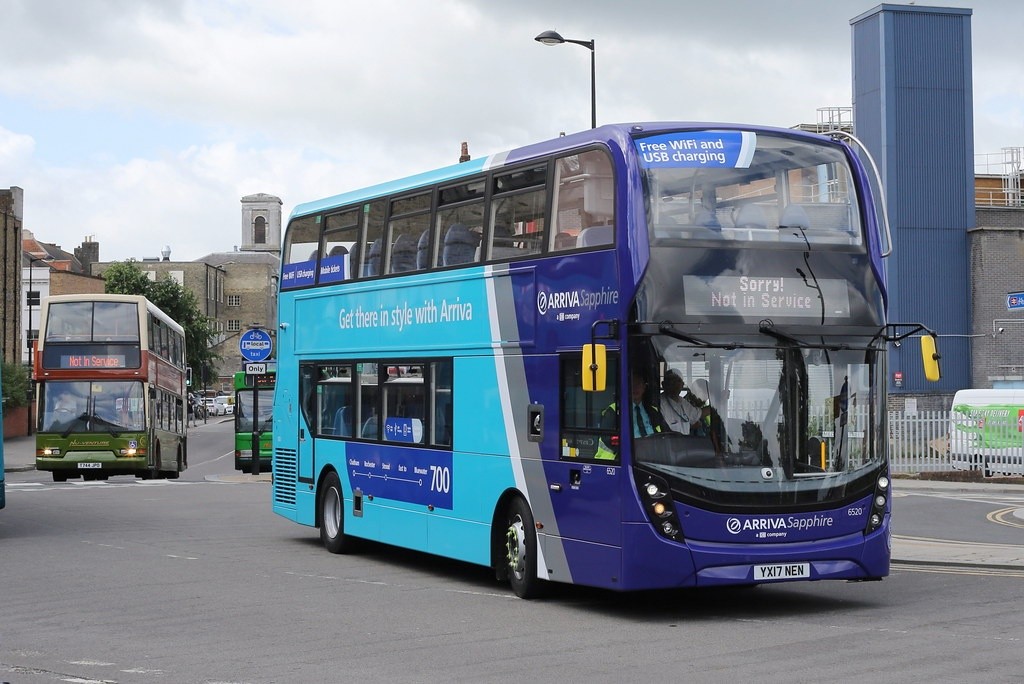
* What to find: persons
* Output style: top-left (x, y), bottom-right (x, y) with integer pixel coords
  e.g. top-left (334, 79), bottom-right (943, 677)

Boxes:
top-left (660, 368), bottom-right (730, 435)
top-left (54, 391), bottom-right (77, 415)
top-left (601, 370), bottom-right (662, 438)
top-left (683, 379), bottom-right (710, 425)
top-left (187, 396), bottom-right (197, 428)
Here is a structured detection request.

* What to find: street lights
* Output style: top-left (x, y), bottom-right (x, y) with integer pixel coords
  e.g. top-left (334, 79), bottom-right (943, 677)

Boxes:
top-left (534, 28), bottom-right (598, 134)
top-left (27, 255), bottom-right (57, 436)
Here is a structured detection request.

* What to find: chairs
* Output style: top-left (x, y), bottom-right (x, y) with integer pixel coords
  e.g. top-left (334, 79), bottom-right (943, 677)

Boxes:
top-left (328, 403), bottom-right (379, 441)
top-left (302, 201), bottom-right (809, 280)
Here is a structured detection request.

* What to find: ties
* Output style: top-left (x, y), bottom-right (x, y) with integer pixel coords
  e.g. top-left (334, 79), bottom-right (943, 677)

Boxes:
top-left (635, 406), bottom-right (647, 437)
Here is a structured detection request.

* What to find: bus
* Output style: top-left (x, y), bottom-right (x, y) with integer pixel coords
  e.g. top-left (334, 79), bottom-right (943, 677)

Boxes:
top-left (234, 360), bottom-right (279, 474)
top-left (37, 293), bottom-right (188, 479)
top-left (269, 118), bottom-right (941, 602)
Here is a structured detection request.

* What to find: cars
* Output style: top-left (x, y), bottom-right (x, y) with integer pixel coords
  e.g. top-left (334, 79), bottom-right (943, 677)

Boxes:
top-left (214, 396), bottom-right (235, 415)
top-left (200, 398), bottom-right (227, 416)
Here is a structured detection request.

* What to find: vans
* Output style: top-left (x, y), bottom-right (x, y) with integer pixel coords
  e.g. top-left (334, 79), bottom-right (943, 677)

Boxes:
top-left (951, 388), bottom-right (1024, 479)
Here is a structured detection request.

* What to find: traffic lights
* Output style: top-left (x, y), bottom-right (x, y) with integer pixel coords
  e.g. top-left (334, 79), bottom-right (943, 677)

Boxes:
top-left (186, 367), bottom-right (192, 386)
